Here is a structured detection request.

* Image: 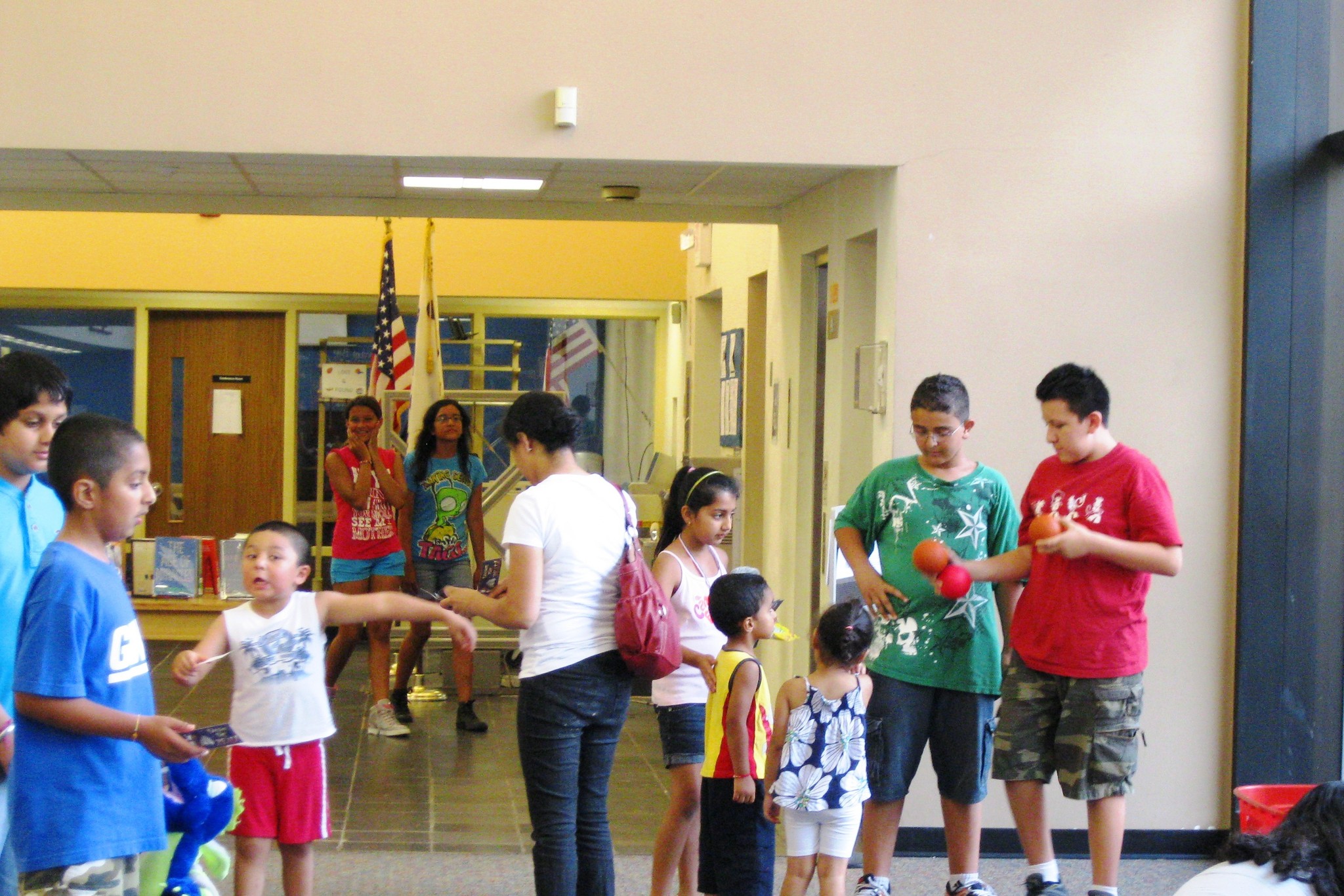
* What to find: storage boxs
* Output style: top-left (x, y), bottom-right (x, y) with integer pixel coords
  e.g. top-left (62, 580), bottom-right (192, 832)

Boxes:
top-left (1232, 781), bottom-right (1317, 835)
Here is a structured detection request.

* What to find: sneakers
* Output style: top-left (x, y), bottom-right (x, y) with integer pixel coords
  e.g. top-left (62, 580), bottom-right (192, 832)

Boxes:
top-left (945, 880), bottom-right (996, 896)
top-left (326, 686), bottom-right (338, 709)
top-left (853, 873), bottom-right (891, 896)
top-left (366, 699), bottom-right (411, 736)
top-left (1025, 873), bottom-right (1069, 896)
top-left (456, 699), bottom-right (488, 730)
top-left (390, 689), bottom-right (413, 722)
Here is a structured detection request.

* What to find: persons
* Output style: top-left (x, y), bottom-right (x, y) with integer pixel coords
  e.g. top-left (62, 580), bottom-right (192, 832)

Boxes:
top-left (1167, 779), bottom-right (1344, 896)
top-left (929, 364), bottom-right (1184, 896)
top-left (388, 398), bottom-right (489, 734)
top-left (762, 596), bottom-right (875, 896)
top-left (565, 393), bottom-right (603, 456)
top-left (5, 414), bottom-right (212, 896)
top-left (0, 350), bottom-right (77, 896)
top-left (438, 389), bottom-right (640, 896)
top-left (649, 466), bottom-right (741, 896)
top-left (170, 518), bottom-right (479, 896)
top-left (696, 570), bottom-right (778, 896)
top-left (324, 394), bottom-right (413, 738)
top-left (833, 373), bottom-right (1030, 896)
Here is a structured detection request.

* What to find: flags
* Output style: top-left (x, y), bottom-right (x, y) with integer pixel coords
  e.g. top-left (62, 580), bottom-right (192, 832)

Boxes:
top-left (405, 220), bottom-right (446, 455)
top-left (365, 223), bottom-right (414, 436)
top-left (540, 313), bottom-right (606, 413)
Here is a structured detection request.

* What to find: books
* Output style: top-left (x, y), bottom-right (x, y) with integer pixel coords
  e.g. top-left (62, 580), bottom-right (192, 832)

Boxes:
top-left (105, 531), bottom-right (256, 601)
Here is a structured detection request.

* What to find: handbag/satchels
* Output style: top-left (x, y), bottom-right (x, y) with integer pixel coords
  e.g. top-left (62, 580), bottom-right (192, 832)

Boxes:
top-left (614, 555), bottom-right (683, 680)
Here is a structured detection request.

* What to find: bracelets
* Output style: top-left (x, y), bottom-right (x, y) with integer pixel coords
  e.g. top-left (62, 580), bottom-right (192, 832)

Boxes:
top-left (0, 721), bottom-right (16, 743)
top-left (360, 459), bottom-right (372, 466)
top-left (0, 718), bottom-right (14, 734)
top-left (131, 714), bottom-right (142, 743)
top-left (732, 772), bottom-right (751, 779)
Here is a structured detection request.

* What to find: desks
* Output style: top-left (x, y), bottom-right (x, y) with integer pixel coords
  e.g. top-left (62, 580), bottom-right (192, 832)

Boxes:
top-left (127, 588), bottom-right (251, 646)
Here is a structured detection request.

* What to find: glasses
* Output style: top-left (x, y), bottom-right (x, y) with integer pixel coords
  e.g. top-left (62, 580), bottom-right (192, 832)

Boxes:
top-left (435, 415), bottom-right (463, 423)
